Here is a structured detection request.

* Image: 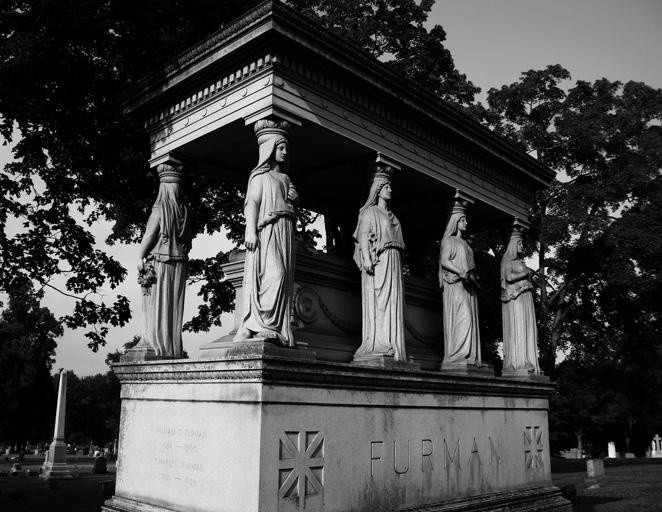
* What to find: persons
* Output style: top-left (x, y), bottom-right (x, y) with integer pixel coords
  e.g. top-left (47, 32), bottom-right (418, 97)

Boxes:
top-left (500, 237), bottom-right (540, 375)
top-left (233, 133), bottom-right (299, 347)
top-left (438, 212), bottom-right (482, 367)
top-left (136, 183), bottom-right (192, 359)
top-left (353, 178), bottom-right (407, 361)
